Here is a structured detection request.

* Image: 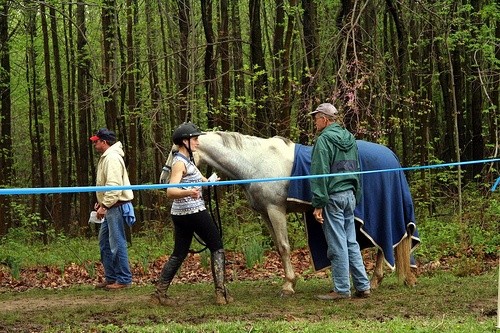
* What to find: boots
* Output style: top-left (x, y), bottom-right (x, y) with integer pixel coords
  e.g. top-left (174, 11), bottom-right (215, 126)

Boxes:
top-left (212, 250), bottom-right (234, 305)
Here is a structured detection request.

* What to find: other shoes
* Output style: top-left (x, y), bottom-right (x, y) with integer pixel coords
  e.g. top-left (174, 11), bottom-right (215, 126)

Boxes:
top-left (105, 282), bottom-right (125, 289)
top-left (151, 254), bottom-right (183, 306)
top-left (96, 282), bottom-right (112, 289)
top-left (352, 290), bottom-right (370, 298)
top-left (317, 291), bottom-right (351, 299)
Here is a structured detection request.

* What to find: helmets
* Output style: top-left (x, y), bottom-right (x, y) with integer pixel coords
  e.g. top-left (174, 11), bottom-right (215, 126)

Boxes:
top-left (175, 123), bottom-right (206, 141)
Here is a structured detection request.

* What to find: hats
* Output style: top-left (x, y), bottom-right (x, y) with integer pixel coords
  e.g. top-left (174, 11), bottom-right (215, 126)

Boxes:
top-left (309, 103), bottom-right (338, 117)
top-left (90, 129), bottom-right (115, 142)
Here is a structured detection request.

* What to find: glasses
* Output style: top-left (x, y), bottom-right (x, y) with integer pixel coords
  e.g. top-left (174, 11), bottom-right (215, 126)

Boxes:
top-left (314, 117), bottom-right (323, 122)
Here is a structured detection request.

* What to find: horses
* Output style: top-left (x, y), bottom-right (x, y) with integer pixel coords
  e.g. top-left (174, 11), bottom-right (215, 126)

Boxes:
top-left (160, 129), bottom-right (413, 297)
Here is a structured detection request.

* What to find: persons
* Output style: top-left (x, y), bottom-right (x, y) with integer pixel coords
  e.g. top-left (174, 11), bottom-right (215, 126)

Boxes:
top-left (147, 125), bottom-right (233, 306)
top-left (308, 103), bottom-right (371, 301)
top-left (90, 128), bottom-right (133, 289)
